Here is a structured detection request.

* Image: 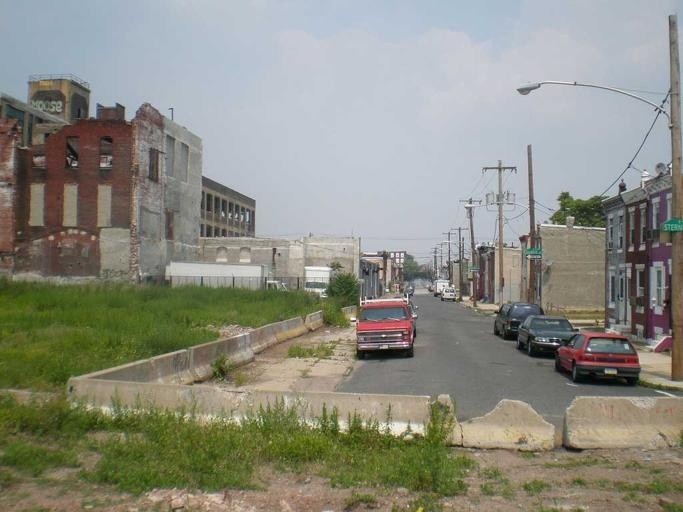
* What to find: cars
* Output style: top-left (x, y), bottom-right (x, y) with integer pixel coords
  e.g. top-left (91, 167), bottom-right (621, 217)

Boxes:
top-left (428, 280), bottom-right (457, 301)
top-left (356, 285), bottom-right (419, 358)
top-left (494, 302), bottom-right (641, 385)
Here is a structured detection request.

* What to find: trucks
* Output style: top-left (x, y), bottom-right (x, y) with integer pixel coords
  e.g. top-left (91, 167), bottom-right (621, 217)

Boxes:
top-left (165, 261), bottom-right (332, 299)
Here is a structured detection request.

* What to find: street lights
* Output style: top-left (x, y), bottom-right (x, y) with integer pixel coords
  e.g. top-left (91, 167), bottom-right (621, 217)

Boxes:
top-left (517, 14), bottom-right (683, 381)
top-left (465, 145), bottom-right (536, 303)
top-left (443, 227), bottom-right (464, 300)
